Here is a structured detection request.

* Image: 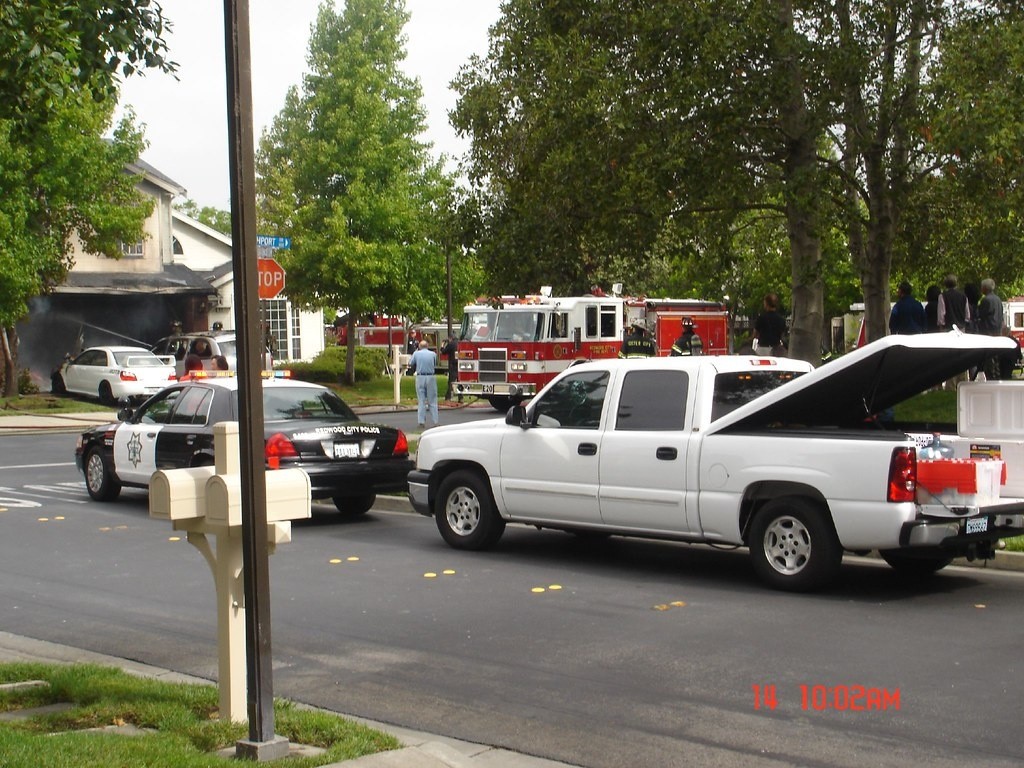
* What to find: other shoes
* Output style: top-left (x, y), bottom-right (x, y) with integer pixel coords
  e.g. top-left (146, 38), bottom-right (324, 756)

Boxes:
top-left (458, 399), bottom-right (464, 404)
top-left (418, 424), bottom-right (425, 429)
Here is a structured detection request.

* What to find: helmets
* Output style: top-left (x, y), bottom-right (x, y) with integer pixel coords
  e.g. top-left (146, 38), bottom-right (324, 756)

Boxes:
top-left (680, 318), bottom-right (694, 326)
top-left (631, 319), bottom-right (646, 330)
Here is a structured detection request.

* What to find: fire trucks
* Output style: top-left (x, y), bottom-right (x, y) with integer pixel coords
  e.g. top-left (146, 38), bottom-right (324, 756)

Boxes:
top-left (451, 287), bottom-right (727, 414)
top-left (335, 314), bottom-right (462, 370)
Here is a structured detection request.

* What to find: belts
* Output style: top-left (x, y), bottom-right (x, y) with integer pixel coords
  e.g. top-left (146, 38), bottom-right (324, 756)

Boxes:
top-left (417, 373), bottom-right (434, 375)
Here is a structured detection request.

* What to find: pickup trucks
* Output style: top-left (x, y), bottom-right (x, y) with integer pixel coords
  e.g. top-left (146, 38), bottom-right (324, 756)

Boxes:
top-left (406, 325), bottom-right (1024, 595)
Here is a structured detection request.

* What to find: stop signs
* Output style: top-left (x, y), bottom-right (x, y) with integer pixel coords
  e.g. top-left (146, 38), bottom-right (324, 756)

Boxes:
top-left (257, 259), bottom-right (286, 299)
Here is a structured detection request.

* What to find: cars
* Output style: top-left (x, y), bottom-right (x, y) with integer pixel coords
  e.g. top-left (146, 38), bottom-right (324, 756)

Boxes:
top-left (76, 371), bottom-right (416, 516)
top-left (51, 345), bottom-right (181, 408)
top-left (148, 330), bottom-right (274, 380)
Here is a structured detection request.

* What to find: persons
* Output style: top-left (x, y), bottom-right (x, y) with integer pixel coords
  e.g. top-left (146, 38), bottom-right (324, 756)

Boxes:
top-left (748, 293), bottom-right (791, 355)
top-left (179, 339), bottom-right (229, 382)
top-left (670, 315), bottom-right (705, 356)
top-left (441, 334), bottom-right (465, 403)
top-left (406, 330), bottom-right (420, 354)
top-left (617, 317), bottom-right (658, 359)
top-left (888, 268), bottom-right (1005, 334)
top-left (407, 339), bottom-right (440, 426)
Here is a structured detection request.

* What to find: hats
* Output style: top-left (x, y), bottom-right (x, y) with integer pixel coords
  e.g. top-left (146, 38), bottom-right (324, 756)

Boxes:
top-left (410, 329), bottom-right (416, 333)
top-left (898, 281), bottom-right (912, 294)
